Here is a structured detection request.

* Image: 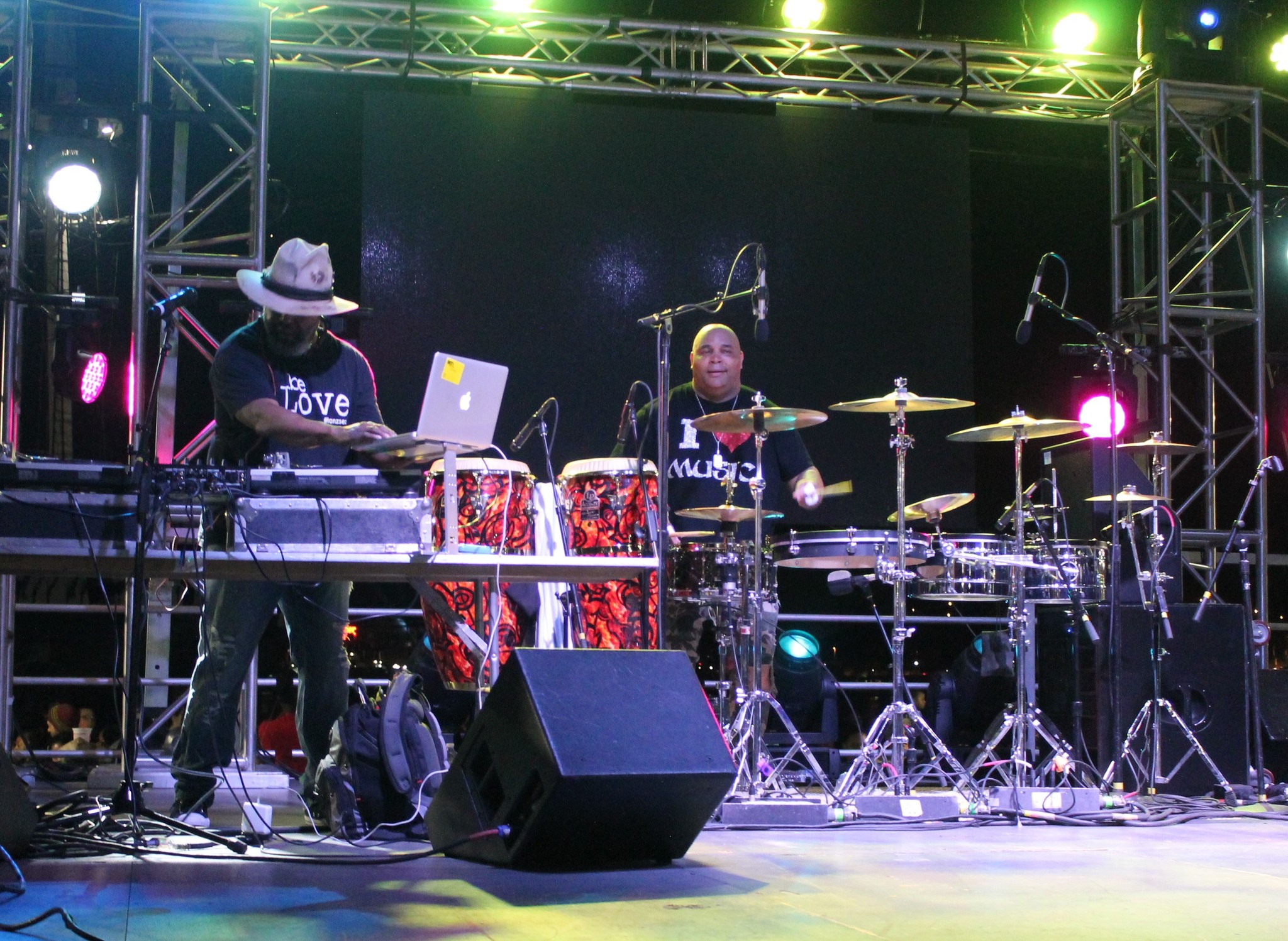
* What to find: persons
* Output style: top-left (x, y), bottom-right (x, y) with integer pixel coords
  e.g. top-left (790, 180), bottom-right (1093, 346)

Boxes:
top-left (259, 671), bottom-right (308, 775)
top-left (14, 703), bottom-right (118, 767)
top-left (845, 690), bottom-right (924, 772)
top-left (170, 238), bottom-right (396, 831)
top-left (611, 324), bottom-right (824, 740)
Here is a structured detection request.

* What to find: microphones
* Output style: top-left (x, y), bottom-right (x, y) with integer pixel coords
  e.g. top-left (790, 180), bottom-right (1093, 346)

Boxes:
top-left (618, 385), bottom-right (636, 444)
top-left (994, 479), bottom-right (1042, 531)
top-left (754, 248), bottom-right (769, 343)
top-left (147, 287), bottom-right (197, 319)
top-left (510, 398), bottom-right (552, 453)
top-left (1017, 254), bottom-right (1047, 344)
top-left (1100, 507), bottom-right (1162, 538)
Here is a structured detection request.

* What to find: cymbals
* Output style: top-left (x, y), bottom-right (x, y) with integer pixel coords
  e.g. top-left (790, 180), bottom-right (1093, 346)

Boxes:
top-left (674, 504), bottom-right (785, 523)
top-left (690, 406), bottom-right (828, 433)
top-left (1083, 493), bottom-right (1175, 501)
top-left (828, 391), bottom-right (975, 413)
top-left (1108, 438), bottom-right (1208, 455)
top-left (887, 492), bottom-right (976, 522)
top-left (945, 416), bottom-right (1092, 443)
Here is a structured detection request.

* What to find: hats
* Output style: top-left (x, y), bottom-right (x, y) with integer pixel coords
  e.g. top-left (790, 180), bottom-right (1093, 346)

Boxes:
top-left (236, 237), bottom-right (359, 317)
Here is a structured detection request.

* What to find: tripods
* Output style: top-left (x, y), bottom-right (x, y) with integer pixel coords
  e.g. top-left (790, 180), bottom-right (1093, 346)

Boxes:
top-left (39, 313), bottom-right (247, 854)
top-left (720, 402), bottom-right (1238, 806)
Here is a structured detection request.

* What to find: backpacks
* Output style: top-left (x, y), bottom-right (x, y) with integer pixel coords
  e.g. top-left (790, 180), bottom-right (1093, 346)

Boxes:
top-left (315, 672), bottom-right (449, 839)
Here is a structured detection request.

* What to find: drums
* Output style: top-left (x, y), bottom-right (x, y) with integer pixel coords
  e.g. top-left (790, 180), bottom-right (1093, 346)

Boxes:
top-left (666, 543), bottom-right (778, 601)
top-left (770, 528), bottom-right (928, 569)
top-left (421, 456), bottom-right (537, 693)
top-left (908, 532), bottom-right (1016, 602)
top-left (1025, 539), bottom-right (1106, 605)
top-left (559, 457), bottom-right (657, 652)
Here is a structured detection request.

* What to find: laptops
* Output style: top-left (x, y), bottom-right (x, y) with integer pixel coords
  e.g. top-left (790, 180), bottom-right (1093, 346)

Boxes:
top-left (350, 351), bottom-right (509, 454)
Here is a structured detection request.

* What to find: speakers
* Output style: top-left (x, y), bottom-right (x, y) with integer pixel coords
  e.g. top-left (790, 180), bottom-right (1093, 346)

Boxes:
top-left (0, 743), bottom-right (38, 861)
top-left (1038, 437), bottom-right (1288, 800)
top-left (425, 647), bottom-right (737, 872)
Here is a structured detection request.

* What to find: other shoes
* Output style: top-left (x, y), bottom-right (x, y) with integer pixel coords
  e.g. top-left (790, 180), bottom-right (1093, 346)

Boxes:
top-left (304, 814), bottom-right (328, 826)
top-left (764, 775), bottom-right (794, 791)
top-left (176, 799), bottom-right (211, 826)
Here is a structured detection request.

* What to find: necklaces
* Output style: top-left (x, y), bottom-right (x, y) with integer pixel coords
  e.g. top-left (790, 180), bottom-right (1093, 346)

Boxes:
top-left (696, 394), bottom-right (738, 468)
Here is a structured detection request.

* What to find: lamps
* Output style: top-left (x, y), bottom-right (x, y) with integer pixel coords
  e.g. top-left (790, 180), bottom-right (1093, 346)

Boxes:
top-left (406, 631), bottom-right (438, 682)
top-left (776, 628), bottom-right (829, 726)
top-left (952, 632), bottom-right (1018, 692)
top-left (37, 134), bottom-right (104, 216)
top-left (1063, 374), bottom-right (1138, 445)
